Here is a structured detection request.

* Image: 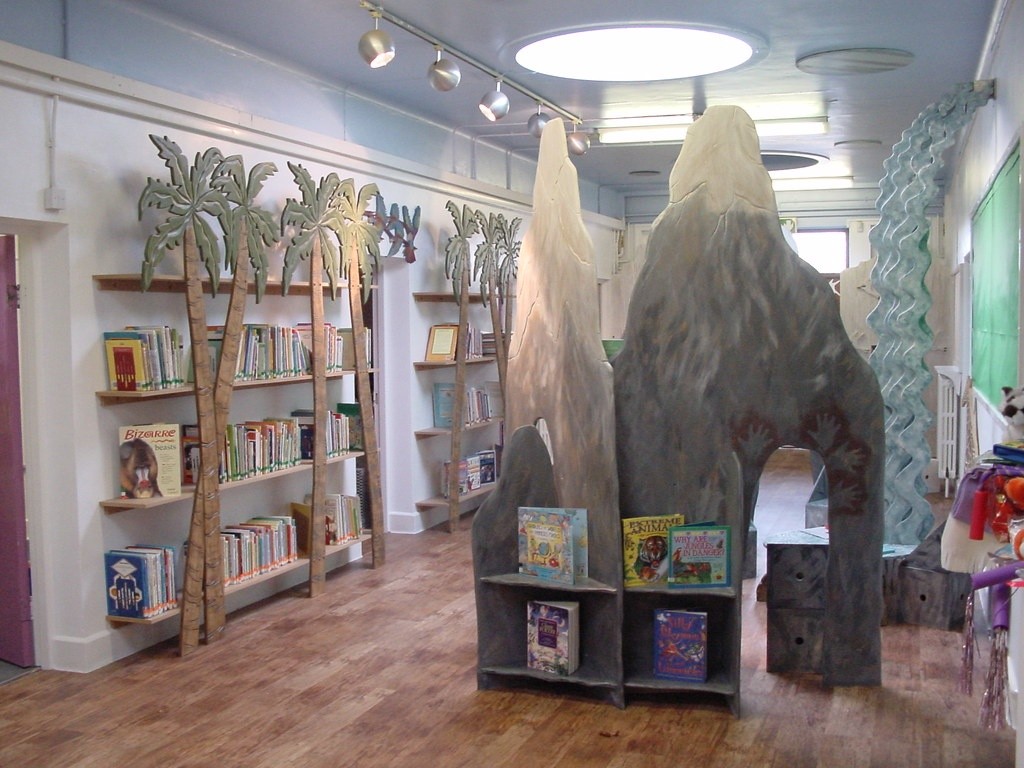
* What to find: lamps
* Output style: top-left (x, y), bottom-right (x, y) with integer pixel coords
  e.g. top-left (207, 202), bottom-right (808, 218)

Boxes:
top-left (358, 1), bottom-right (591, 156)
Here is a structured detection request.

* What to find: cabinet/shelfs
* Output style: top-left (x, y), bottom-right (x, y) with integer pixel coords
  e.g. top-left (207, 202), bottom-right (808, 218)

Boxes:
top-left (410, 290), bottom-right (518, 511)
top-left (90, 274), bottom-right (393, 631)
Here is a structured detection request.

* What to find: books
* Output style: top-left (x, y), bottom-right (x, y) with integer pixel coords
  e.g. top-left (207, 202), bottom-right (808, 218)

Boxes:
top-left (652, 608), bottom-right (708, 682)
top-left (622, 515), bottom-right (732, 590)
top-left (517, 506), bottom-right (590, 586)
top-left (431, 379), bottom-right (493, 429)
top-left (441, 444), bottom-right (500, 498)
top-left (101, 493), bottom-right (364, 618)
top-left (118, 402), bottom-right (363, 500)
top-left (527, 601), bottom-right (580, 676)
top-left (103, 321), bottom-right (373, 392)
top-left (425, 324), bottom-right (515, 362)
